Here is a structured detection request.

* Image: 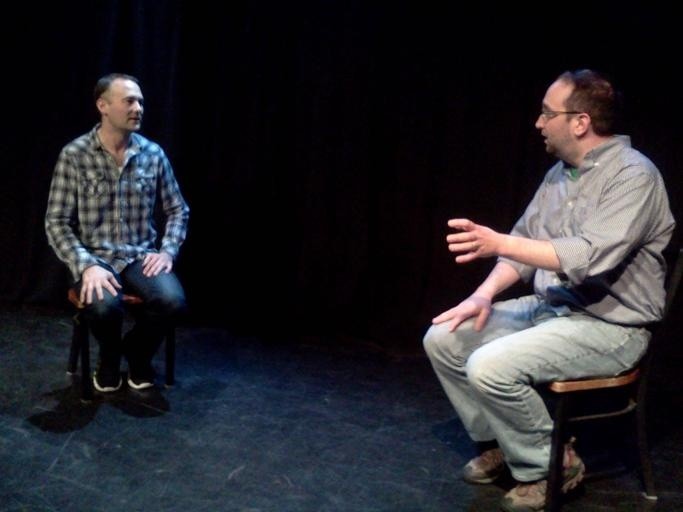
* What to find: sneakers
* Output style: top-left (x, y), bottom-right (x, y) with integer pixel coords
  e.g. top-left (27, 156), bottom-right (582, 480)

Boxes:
top-left (460, 445), bottom-right (507, 486)
top-left (91, 357), bottom-right (123, 392)
top-left (500, 443), bottom-right (586, 510)
top-left (123, 339), bottom-right (156, 388)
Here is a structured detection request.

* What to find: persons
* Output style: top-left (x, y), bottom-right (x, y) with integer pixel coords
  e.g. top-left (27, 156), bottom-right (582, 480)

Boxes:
top-left (422, 67), bottom-right (678, 509)
top-left (41, 73), bottom-right (191, 413)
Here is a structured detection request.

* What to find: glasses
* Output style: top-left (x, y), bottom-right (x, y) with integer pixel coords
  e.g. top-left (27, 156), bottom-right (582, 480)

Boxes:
top-left (540, 109), bottom-right (582, 120)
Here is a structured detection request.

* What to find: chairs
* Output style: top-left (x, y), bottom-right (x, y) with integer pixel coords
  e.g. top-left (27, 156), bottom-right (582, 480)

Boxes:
top-left (68, 189), bottom-right (177, 404)
top-left (547, 241), bottom-right (682, 511)
